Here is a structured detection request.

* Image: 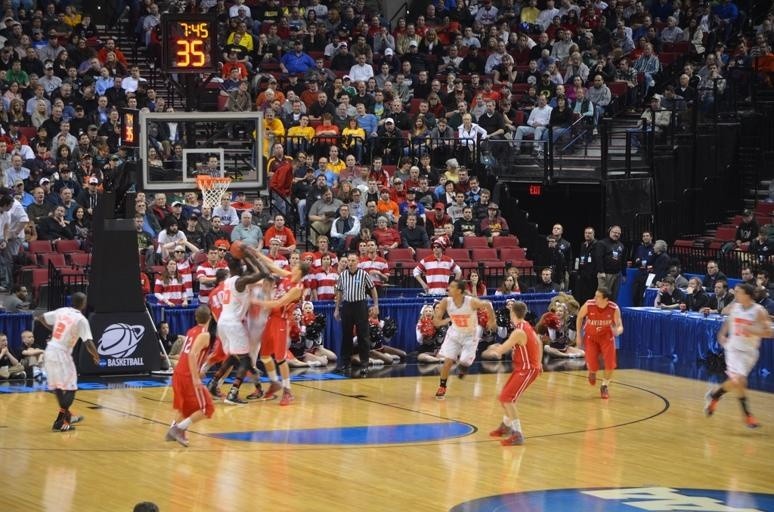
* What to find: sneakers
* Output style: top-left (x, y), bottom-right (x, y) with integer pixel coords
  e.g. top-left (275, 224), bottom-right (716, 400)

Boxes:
top-left (369, 358), bottom-right (385, 365)
top-left (502, 430), bottom-right (524, 445)
top-left (54, 420), bottom-right (74, 431)
top-left (704, 391), bottom-right (717, 414)
top-left (459, 370), bottom-right (465, 378)
top-left (744, 413), bottom-right (758, 428)
top-left (12, 371), bottom-right (27, 379)
top-left (33, 369), bottom-right (43, 378)
top-left (66, 414), bottom-right (84, 423)
top-left (208, 381), bottom-right (294, 405)
top-left (490, 423), bottom-right (509, 435)
top-left (588, 371), bottom-right (596, 386)
top-left (339, 364), bottom-right (369, 374)
top-left (165, 423), bottom-right (188, 446)
top-left (384, 352), bottom-right (400, 360)
top-left (307, 360), bottom-right (321, 367)
top-left (436, 386), bottom-right (446, 396)
top-left (600, 384), bottom-right (609, 398)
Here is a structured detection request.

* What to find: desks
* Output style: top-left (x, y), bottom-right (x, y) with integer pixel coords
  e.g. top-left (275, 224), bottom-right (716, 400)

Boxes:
top-left (620, 307), bottom-right (773, 380)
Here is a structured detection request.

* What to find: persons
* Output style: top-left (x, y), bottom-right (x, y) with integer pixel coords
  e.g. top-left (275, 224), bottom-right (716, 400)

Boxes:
top-left (134, 502), bottom-right (159, 512)
top-left (1, 2), bottom-right (773, 447)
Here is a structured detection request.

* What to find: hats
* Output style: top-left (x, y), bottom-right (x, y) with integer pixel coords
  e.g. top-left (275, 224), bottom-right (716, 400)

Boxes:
top-left (584, 31), bottom-right (594, 39)
top-left (431, 233), bottom-right (451, 250)
top-left (338, 41), bottom-right (348, 48)
top-left (487, 203), bottom-right (498, 209)
top-left (452, 78), bottom-right (463, 85)
top-left (172, 201), bottom-right (199, 219)
top-left (409, 41), bottom-right (418, 47)
top-left (15, 178), bottom-right (23, 186)
top-left (384, 47), bottom-right (393, 56)
top-left (741, 208), bottom-right (751, 216)
top-left (650, 93), bottom-right (662, 102)
top-left (1, 16), bottom-right (58, 70)
top-left (38, 141), bottom-right (119, 184)
top-left (305, 167), bottom-right (315, 172)
top-left (384, 118), bottom-right (393, 124)
top-left (393, 178), bottom-right (417, 207)
top-left (542, 70), bottom-right (551, 78)
top-left (75, 105), bottom-right (98, 130)
top-left (435, 203), bottom-right (445, 210)
top-left (294, 39), bottom-right (302, 45)
top-left (267, 68), bottom-right (352, 83)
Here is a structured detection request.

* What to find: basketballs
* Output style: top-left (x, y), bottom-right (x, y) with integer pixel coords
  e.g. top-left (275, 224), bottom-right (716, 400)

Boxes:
top-left (230, 240), bottom-right (245, 258)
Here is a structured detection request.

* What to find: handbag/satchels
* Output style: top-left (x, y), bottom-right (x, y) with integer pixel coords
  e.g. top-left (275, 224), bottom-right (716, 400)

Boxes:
top-left (24, 221), bottom-right (38, 243)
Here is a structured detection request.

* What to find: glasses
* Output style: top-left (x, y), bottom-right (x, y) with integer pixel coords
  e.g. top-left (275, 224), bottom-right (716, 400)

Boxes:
top-left (176, 250), bottom-right (184, 253)
top-left (219, 248), bottom-right (226, 252)
